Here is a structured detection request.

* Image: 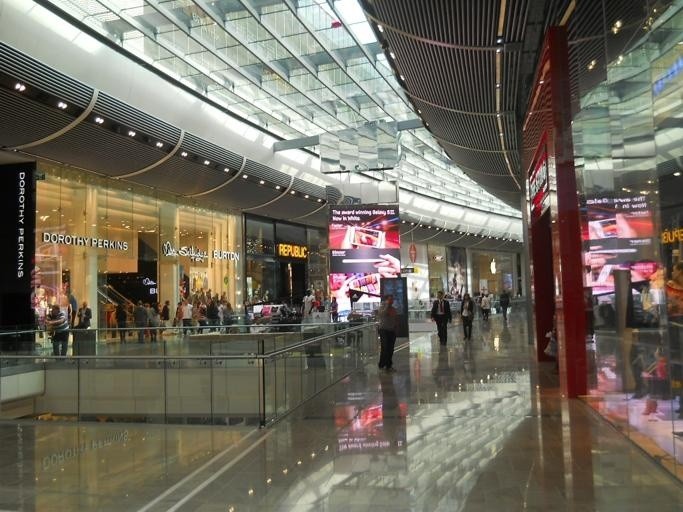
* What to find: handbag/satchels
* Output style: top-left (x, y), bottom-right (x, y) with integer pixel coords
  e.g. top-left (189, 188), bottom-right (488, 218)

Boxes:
top-left (468, 311), bottom-right (474, 321)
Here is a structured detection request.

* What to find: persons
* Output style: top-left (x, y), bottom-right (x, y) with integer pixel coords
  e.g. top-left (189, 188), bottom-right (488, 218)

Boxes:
top-left (480, 293), bottom-right (491, 320)
top-left (461, 294), bottom-right (474, 341)
top-left (45, 305), bottom-right (69, 360)
top-left (77, 301), bottom-right (92, 329)
top-left (303, 282), bottom-right (338, 322)
top-left (378, 295), bottom-right (399, 372)
top-left (115, 300), bottom-right (170, 344)
top-left (499, 290), bottom-right (509, 320)
top-left (430, 291), bottom-right (452, 345)
top-left (176, 287), bottom-right (234, 334)
top-left (341, 225), bottom-right (386, 250)
top-left (373, 253), bottom-right (400, 278)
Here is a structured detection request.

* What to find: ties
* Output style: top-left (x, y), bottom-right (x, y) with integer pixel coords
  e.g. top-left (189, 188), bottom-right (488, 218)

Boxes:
top-left (439, 301), bottom-right (443, 312)
